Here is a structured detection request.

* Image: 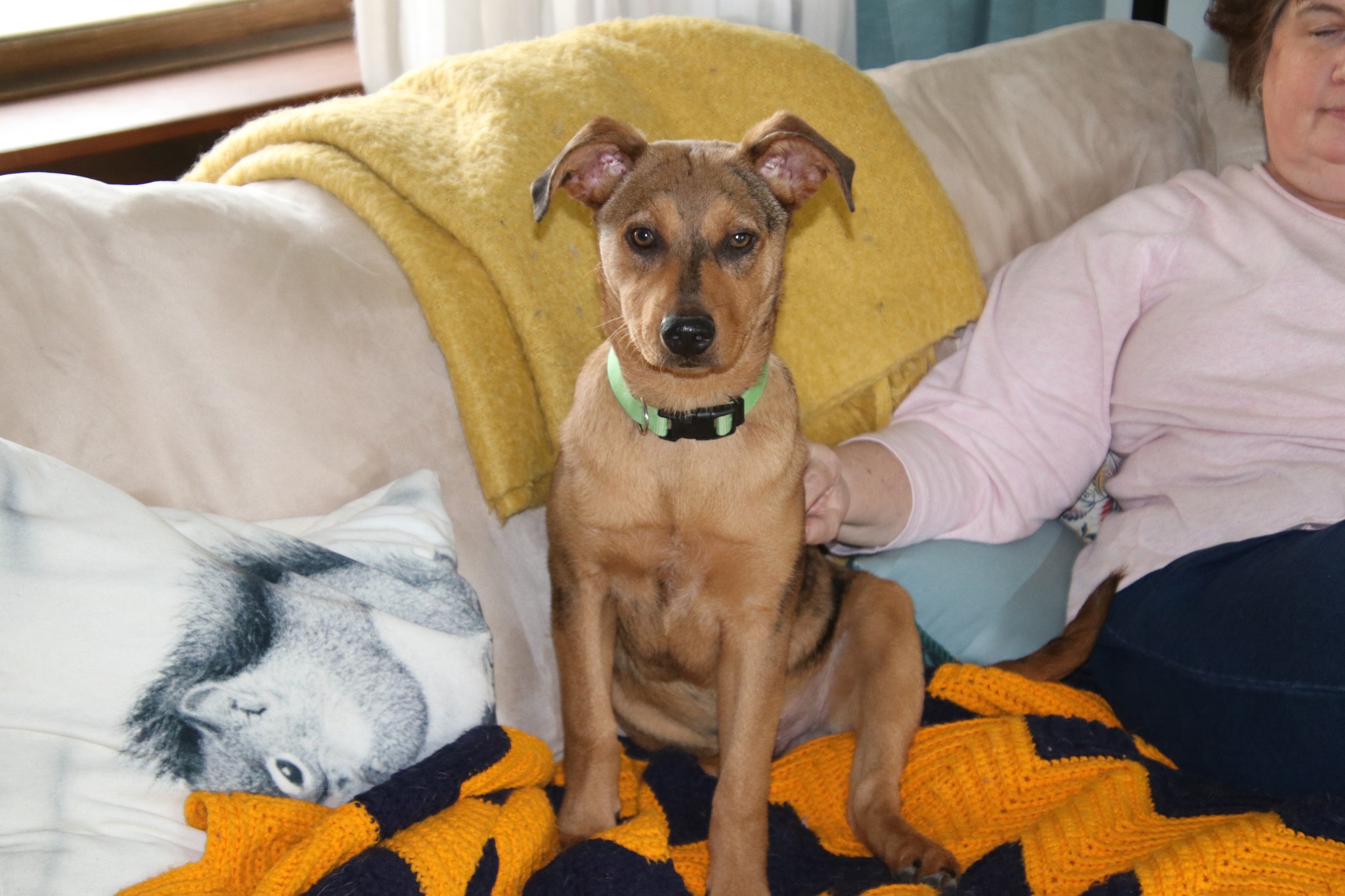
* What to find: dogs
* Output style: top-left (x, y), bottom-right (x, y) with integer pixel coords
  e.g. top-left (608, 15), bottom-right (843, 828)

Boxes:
top-left (532, 115), bottom-right (962, 895)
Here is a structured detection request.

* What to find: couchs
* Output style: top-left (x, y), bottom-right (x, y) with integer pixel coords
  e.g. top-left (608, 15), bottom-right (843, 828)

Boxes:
top-left (0, 17), bottom-right (1345, 896)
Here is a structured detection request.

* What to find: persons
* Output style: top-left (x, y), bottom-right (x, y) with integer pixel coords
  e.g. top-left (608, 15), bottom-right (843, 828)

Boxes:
top-left (803, 0), bottom-right (1345, 831)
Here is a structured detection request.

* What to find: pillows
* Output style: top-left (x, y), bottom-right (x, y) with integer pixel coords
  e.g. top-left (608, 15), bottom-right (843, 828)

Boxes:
top-left (0, 435), bottom-right (496, 896)
